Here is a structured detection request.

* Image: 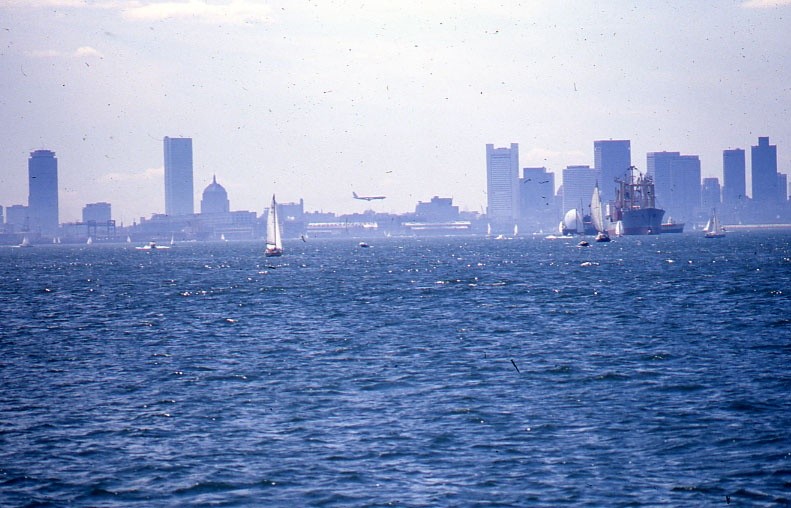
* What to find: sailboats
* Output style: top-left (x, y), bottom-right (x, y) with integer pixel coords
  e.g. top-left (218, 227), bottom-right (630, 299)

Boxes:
top-left (558, 180), bottom-right (607, 235)
top-left (264, 194), bottom-right (283, 256)
top-left (577, 199), bottom-right (589, 246)
top-left (170, 232), bottom-right (176, 246)
top-left (19, 236), bottom-right (131, 247)
top-left (703, 208), bottom-right (728, 238)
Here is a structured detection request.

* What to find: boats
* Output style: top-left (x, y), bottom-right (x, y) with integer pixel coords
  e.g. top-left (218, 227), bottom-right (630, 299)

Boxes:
top-left (607, 165), bottom-right (666, 235)
top-left (595, 232), bottom-right (611, 243)
top-left (660, 217), bottom-right (685, 232)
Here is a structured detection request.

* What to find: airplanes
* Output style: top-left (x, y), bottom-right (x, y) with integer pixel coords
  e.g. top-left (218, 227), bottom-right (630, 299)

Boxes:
top-left (352, 192), bottom-right (386, 201)
